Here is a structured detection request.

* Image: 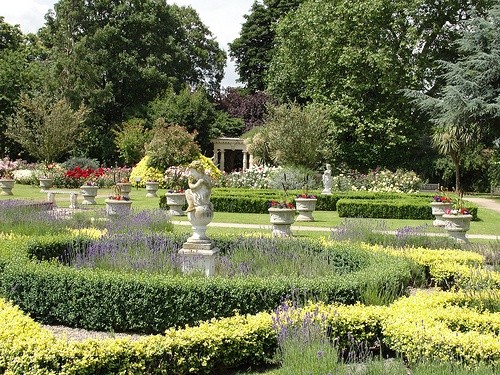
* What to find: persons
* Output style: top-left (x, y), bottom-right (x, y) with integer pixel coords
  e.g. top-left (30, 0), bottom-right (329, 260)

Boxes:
top-left (184, 161), bottom-right (213, 213)
top-left (322, 164), bottom-right (332, 193)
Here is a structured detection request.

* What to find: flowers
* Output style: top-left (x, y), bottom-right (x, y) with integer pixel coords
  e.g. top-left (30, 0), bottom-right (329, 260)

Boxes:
top-left (109, 194), bottom-right (129, 201)
top-left (443, 206), bottom-right (471, 215)
top-left (168, 189), bottom-right (184, 193)
top-left (433, 195), bottom-right (451, 203)
top-left (85, 181), bottom-right (99, 186)
top-left (269, 200), bottom-right (294, 208)
top-left (296, 193), bottom-right (317, 199)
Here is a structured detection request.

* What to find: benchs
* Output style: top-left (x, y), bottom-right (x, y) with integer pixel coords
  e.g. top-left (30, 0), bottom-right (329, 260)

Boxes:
top-left (41, 189), bottom-right (81, 208)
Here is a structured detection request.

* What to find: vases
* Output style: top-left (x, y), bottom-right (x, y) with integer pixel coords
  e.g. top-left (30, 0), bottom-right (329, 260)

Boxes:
top-left (165, 193), bottom-right (186, 215)
top-left (442, 215), bottom-right (473, 244)
top-left (81, 186), bottom-right (98, 205)
top-left (295, 198), bottom-right (317, 222)
top-left (106, 199), bottom-right (133, 215)
top-left (431, 202), bottom-right (453, 225)
top-left (268, 207), bottom-right (297, 240)
